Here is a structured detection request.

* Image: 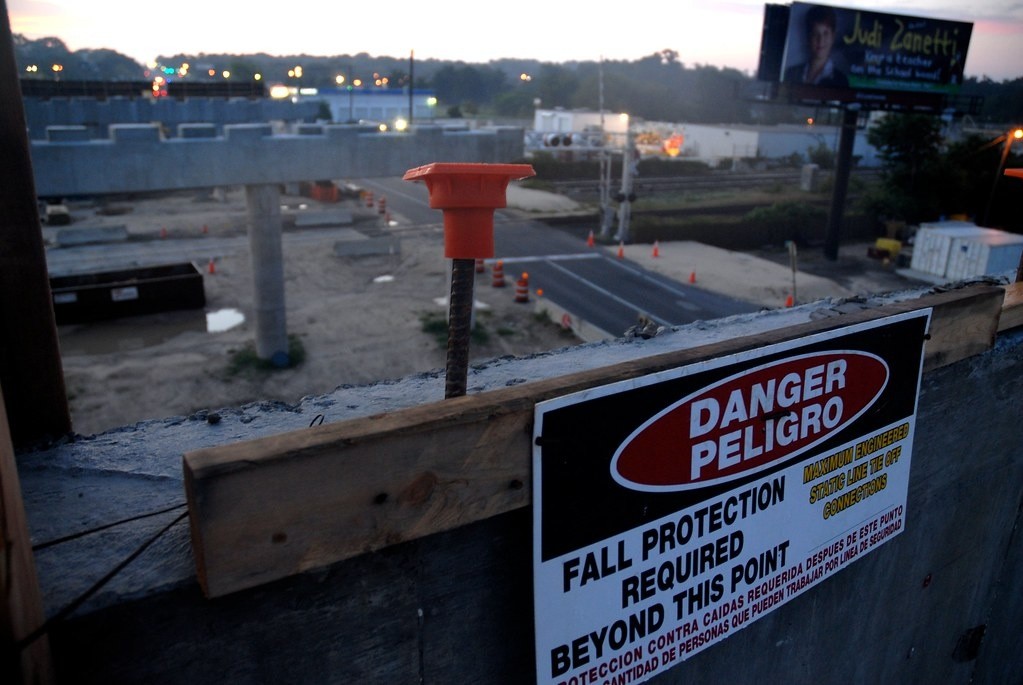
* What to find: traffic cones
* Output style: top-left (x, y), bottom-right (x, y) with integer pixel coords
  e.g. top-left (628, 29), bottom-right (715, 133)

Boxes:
top-left (161, 228), bottom-right (168, 239)
top-left (653, 240), bottom-right (661, 256)
top-left (209, 258), bottom-right (218, 273)
top-left (688, 267), bottom-right (697, 283)
top-left (202, 224), bottom-right (209, 233)
top-left (617, 240), bottom-right (625, 257)
top-left (587, 230), bottom-right (597, 248)
top-left (786, 294), bottom-right (795, 308)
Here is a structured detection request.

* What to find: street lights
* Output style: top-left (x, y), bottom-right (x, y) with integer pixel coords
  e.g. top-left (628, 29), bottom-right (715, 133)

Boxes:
top-left (336, 65), bottom-right (365, 123)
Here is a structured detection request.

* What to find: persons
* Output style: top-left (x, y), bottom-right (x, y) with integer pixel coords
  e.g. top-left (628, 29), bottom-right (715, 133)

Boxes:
top-left (789, 5), bottom-right (849, 81)
